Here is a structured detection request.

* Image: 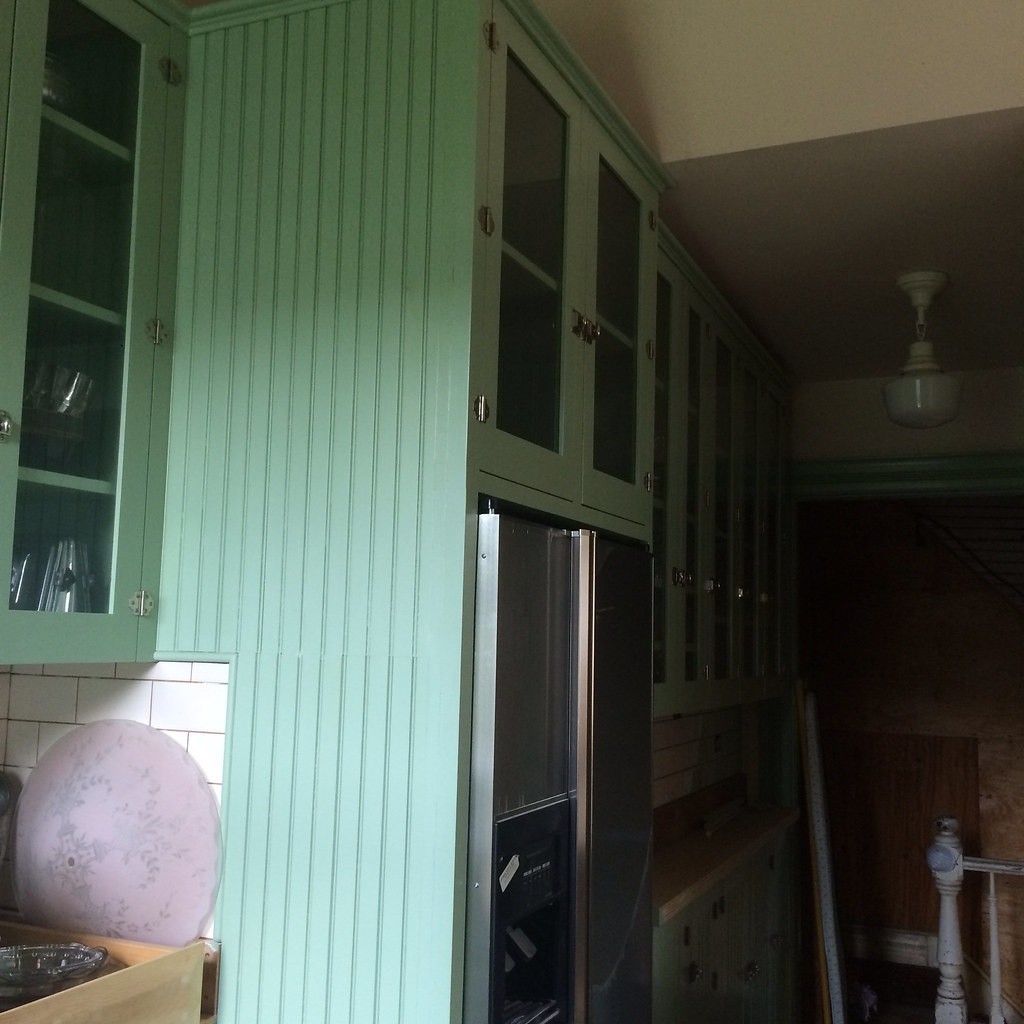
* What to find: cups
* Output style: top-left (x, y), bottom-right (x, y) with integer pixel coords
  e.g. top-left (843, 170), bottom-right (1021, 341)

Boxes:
top-left (43, 49), bottom-right (74, 114)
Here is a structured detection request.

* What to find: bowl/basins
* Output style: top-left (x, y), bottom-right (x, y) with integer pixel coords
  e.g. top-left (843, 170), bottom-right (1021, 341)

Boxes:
top-left (0, 941), bottom-right (108, 997)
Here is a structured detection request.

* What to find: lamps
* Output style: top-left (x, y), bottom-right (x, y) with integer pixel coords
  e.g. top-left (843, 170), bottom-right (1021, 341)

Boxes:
top-left (885, 268), bottom-right (962, 427)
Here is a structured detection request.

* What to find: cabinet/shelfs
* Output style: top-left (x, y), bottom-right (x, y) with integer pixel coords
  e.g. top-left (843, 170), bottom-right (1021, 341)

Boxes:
top-left (649, 219), bottom-right (800, 721)
top-left (153, 3), bottom-right (683, 1022)
top-left (0, 920), bottom-right (205, 1024)
top-left (654, 807), bottom-right (808, 1024)
top-left (0, 1), bottom-right (193, 663)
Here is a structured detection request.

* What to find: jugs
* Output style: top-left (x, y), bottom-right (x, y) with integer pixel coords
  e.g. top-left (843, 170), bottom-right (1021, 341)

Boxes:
top-left (14, 520), bottom-right (93, 613)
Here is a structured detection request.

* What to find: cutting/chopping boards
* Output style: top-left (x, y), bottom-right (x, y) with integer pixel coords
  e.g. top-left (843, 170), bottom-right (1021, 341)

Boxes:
top-left (9, 714), bottom-right (226, 950)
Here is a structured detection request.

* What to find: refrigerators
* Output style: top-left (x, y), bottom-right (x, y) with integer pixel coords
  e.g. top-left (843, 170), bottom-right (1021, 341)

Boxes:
top-left (464, 506), bottom-right (655, 1024)
